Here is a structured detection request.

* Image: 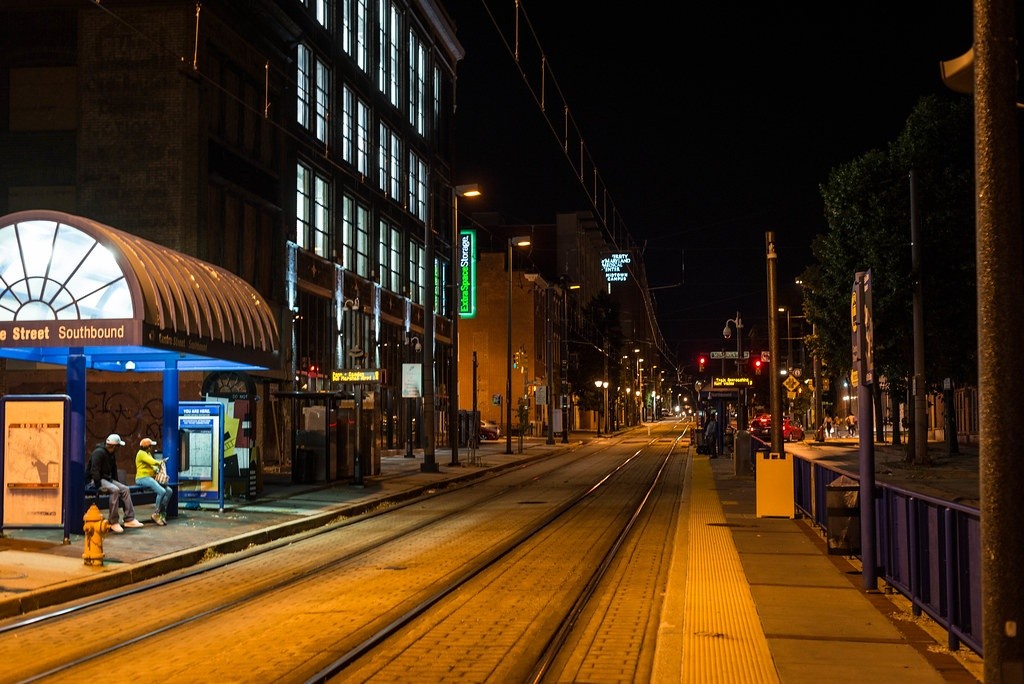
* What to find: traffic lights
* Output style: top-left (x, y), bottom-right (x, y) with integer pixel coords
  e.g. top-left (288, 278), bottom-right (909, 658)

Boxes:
top-left (700, 357), bottom-right (705, 372)
top-left (756, 360), bottom-right (761, 375)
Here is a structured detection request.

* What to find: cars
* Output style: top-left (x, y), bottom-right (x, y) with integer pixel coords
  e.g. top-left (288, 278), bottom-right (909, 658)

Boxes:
top-left (749, 418), bottom-right (806, 443)
top-left (480, 420), bottom-right (501, 440)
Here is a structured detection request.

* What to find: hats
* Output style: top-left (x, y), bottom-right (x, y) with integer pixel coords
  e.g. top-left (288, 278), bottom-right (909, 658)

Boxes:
top-left (140, 438), bottom-right (157, 447)
top-left (106, 434), bottom-right (126, 446)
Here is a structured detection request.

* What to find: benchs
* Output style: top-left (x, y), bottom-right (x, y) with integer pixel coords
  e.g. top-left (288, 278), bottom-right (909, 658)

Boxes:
top-left (84, 484), bottom-right (158, 510)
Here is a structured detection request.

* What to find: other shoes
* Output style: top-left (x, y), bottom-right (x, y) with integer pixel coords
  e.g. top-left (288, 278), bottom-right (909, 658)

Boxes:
top-left (160, 513), bottom-right (167, 525)
top-left (711, 455), bottom-right (718, 459)
top-left (111, 523), bottom-right (124, 533)
top-left (124, 518), bottom-right (144, 527)
top-left (151, 513), bottom-right (164, 526)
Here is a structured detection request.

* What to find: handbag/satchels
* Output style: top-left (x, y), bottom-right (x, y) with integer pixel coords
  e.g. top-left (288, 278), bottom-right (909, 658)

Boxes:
top-left (155, 462), bottom-right (169, 485)
top-left (697, 443), bottom-right (710, 455)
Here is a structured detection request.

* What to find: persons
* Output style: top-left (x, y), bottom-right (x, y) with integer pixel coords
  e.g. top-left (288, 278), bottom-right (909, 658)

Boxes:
top-left (86, 434), bottom-right (144, 533)
top-left (824, 414), bottom-right (858, 437)
top-left (135, 438), bottom-right (173, 526)
top-left (704, 415), bottom-right (717, 459)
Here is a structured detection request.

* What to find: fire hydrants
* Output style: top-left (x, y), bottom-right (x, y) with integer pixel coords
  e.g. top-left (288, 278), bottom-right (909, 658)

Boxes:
top-left (82, 501), bottom-right (112, 566)
top-left (817, 426), bottom-right (825, 442)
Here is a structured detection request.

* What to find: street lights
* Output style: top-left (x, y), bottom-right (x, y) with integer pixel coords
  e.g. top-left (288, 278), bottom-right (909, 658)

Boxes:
top-left (795, 276), bottom-right (823, 430)
top-left (447, 183), bottom-right (481, 468)
top-left (595, 379), bottom-right (609, 435)
top-left (503, 236), bottom-right (530, 454)
top-left (625, 388), bottom-right (660, 426)
top-left (636, 358), bottom-right (644, 380)
top-left (559, 284), bottom-right (582, 443)
top-left (650, 365), bottom-right (656, 379)
top-left (778, 305), bottom-right (793, 371)
